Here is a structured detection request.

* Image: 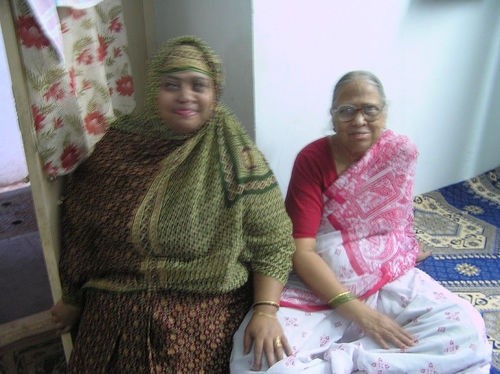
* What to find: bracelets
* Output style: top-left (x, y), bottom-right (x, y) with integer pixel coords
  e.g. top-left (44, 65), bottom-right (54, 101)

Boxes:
top-left (327, 291), bottom-right (354, 309)
top-left (253, 311), bottom-right (277, 320)
top-left (252, 301), bottom-right (280, 309)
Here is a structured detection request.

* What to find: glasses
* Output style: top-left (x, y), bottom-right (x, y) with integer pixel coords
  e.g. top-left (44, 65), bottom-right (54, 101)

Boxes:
top-left (330, 101), bottom-right (385, 123)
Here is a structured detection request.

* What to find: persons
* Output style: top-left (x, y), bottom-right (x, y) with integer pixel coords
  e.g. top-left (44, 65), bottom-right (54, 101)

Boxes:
top-left (229, 72), bottom-right (493, 374)
top-left (51, 36), bottom-right (296, 374)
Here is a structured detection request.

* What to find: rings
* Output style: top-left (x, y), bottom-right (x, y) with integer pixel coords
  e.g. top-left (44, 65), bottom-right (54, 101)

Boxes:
top-left (274, 338), bottom-right (282, 347)
